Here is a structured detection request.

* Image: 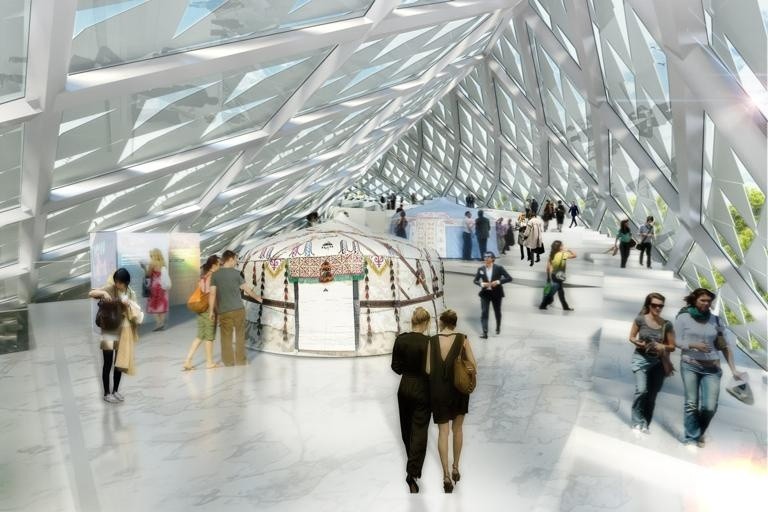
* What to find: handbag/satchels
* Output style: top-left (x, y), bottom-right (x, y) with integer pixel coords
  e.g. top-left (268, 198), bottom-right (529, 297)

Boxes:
top-left (95, 300), bottom-right (123, 329)
top-left (630, 238), bottom-right (636, 247)
top-left (454, 358), bottom-right (476, 392)
top-left (188, 287), bottom-right (209, 314)
top-left (160, 265), bottom-right (172, 289)
top-left (636, 244), bottom-right (642, 250)
top-left (142, 277), bottom-right (151, 298)
top-left (552, 269), bottom-right (567, 282)
top-left (715, 334), bottom-right (728, 351)
top-left (661, 349), bottom-right (674, 376)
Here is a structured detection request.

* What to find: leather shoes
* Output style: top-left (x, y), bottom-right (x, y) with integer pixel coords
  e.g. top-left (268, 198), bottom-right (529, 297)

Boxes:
top-left (406, 475), bottom-right (419, 493)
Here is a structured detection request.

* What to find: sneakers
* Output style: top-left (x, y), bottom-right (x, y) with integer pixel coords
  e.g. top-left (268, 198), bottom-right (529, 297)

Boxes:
top-left (104, 394), bottom-right (119, 403)
top-left (152, 323), bottom-right (164, 331)
top-left (114, 392), bottom-right (124, 401)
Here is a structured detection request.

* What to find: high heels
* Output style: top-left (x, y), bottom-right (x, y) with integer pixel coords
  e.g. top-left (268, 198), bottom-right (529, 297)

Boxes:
top-left (444, 476), bottom-right (453, 493)
top-left (452, 466), bottom-right (460, 485)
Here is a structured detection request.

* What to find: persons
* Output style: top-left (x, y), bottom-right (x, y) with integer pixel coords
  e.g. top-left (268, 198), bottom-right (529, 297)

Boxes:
top-left (389, 305), bottom-right (433, 495)
top-left (566, 101), bottom-right (673, 190)
top-left (473, 250), bottom-right (513, 338)
top-left (628, 292), bottom-right (677, 436)
top-left (206, 250), bottom-right (265, 368)
top-left (182, 254), bottom-right (222, 371)
top-left (425, 309), bottom-right (478, 495)
top-left (138, 247), bottom-right (171, 333)
top-left (305, 190), bottom-right (660, 270)
top-left (535, 240), bottom-right (578, 312)
top-left (88, 267), bottom-right (141, 404)
top-left (671, 288), bottom-right (745, 449)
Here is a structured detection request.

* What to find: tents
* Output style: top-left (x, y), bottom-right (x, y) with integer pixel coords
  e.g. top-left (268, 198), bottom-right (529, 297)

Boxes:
top-left (238, 213), bottom-right (445, 358)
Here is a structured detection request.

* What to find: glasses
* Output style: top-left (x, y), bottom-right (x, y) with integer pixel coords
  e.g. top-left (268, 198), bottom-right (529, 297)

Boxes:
top-left (650, 303), bottom-right (665, 308)
